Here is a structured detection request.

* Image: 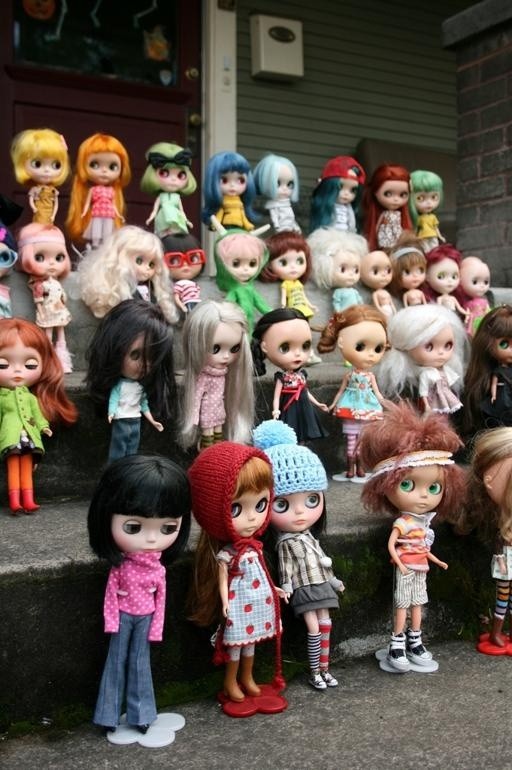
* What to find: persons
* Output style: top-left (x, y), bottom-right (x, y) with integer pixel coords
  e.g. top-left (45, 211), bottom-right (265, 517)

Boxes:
top-left (0, 129), bottom-right (512, 747)
